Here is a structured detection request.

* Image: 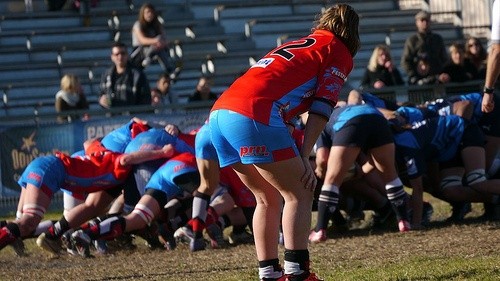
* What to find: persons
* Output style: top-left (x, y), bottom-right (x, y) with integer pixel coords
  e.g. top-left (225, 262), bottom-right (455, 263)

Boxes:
top-left (147, 74), bottom-right (171, 103)
top-left (360, 44), bottom-right (405, 108)
top-left (399, 9), bottom-right (445, 84)
top-left (1, 94), bottom-right (499, 261)
top-left (481, 0), bottom-right (499, 113)
top-left (127, 4), bottom-right (181, 77)
top-left (408, 49), bottom-right (447, 95)
top-left (440, 41), bottom-right (478, 83)
top-left (466, 36), bottom-right (488, 66)
top-left (95, 43), bottom-right (152, 110)
top-left (54, 71), bottom-right (88, 123)
top-left (208, 3), bottom-right (359, 281)
top-left (188, 75), bottom-right (217, 105)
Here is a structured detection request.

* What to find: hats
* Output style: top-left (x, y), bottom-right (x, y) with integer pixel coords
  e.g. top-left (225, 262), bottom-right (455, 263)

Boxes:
top-left (414, 10), bottom-right (431, 20)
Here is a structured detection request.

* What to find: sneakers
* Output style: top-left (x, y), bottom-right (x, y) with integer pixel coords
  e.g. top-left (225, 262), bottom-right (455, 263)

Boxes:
top-left (446, 202), bottom-right (471, 223)
top-left (397, 221), bottom-right (411, 233)
top-left (188, 238), bottom-right (207, 251)
top-left (328, 222), bottom-right (346, 236)
top-left (261, 273), bottom-right (286, 281)
top-left (103, 238), bottom-right (118, 250)
top-left (421, 204), bottom-right (433, 222)
top-left (365, 214), bottom-right (396, 234)
top-left (286, 272), bottom-right (323, 281)
top-left (308, 228), bottom-right (327, 244)
top-left (61, 230), bottom-right (78, 258)
top-left (70, 231), bottom-right (98, 259)
top-left (173, 227), bottom-right (193, 244)
top-left (207, 223), bottom-right (226, 250)
top-left (227, 232), bottom-right (254, 245)
top-left (151, 222), bottom-right (178, 252)
top-left (9, 237), bottom-right (31, 258)
top-left (35, 231), bottom-right (63, 256)
top-left (114, 234), bottom-right (138, 251)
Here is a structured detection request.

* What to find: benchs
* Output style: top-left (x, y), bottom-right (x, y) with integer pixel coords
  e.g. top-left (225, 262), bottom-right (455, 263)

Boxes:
top-left (0, 1), bottom-right (493, 131)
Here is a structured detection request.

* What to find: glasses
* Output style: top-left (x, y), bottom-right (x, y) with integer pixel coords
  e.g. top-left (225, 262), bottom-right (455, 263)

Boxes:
top-left (468, 43), bottom-right (482, 49)
top-left (111, 51), bottom-right (129, 56)
